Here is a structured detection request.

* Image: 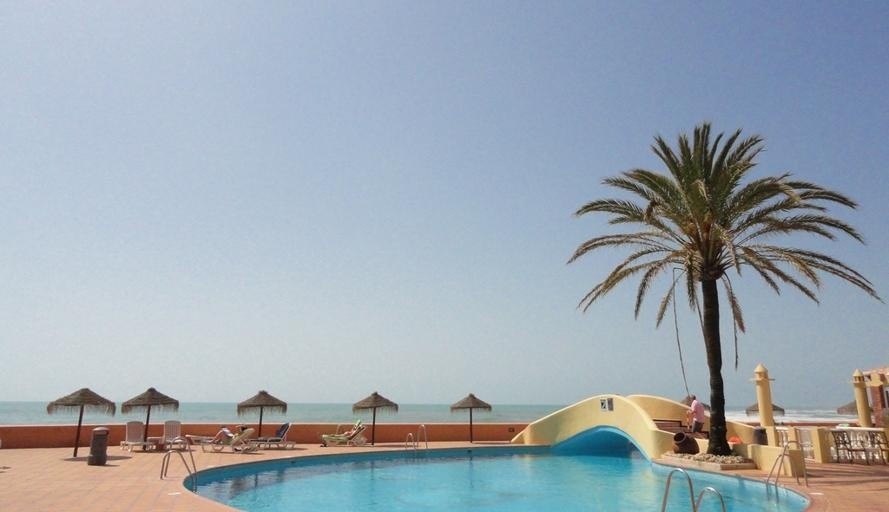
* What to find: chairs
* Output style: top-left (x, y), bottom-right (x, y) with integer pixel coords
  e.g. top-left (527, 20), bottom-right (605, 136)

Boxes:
top-left (829, 428), bottom-right (889, 466)
top-left (120, 418), bottom-right (370, 453)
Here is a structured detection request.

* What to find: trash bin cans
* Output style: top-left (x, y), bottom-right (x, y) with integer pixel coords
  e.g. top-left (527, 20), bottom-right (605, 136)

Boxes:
top-left (87, 427), bottom-right (110, 465)
top-left (753, 427), bottom-right (768, 445)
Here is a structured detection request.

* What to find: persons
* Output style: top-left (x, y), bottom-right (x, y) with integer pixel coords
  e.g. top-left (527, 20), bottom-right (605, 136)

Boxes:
top-left (329, 426), bottom-right (357, 437)
top-left (686, 394), bottom-right (706, 434)
top-left (208, 424), bottom-right (248, 444)
